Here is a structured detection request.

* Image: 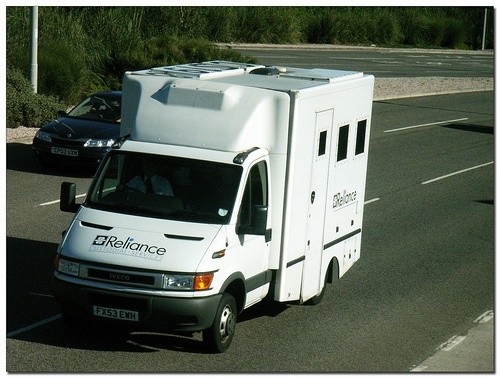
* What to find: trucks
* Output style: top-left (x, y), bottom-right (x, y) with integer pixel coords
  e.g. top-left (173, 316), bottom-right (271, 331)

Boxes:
top-left (53, 62), bottom-right (375, 353)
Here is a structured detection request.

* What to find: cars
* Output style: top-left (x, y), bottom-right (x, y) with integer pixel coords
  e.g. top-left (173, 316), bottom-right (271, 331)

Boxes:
top-left (33, 91), bottom-right (120, 174)
